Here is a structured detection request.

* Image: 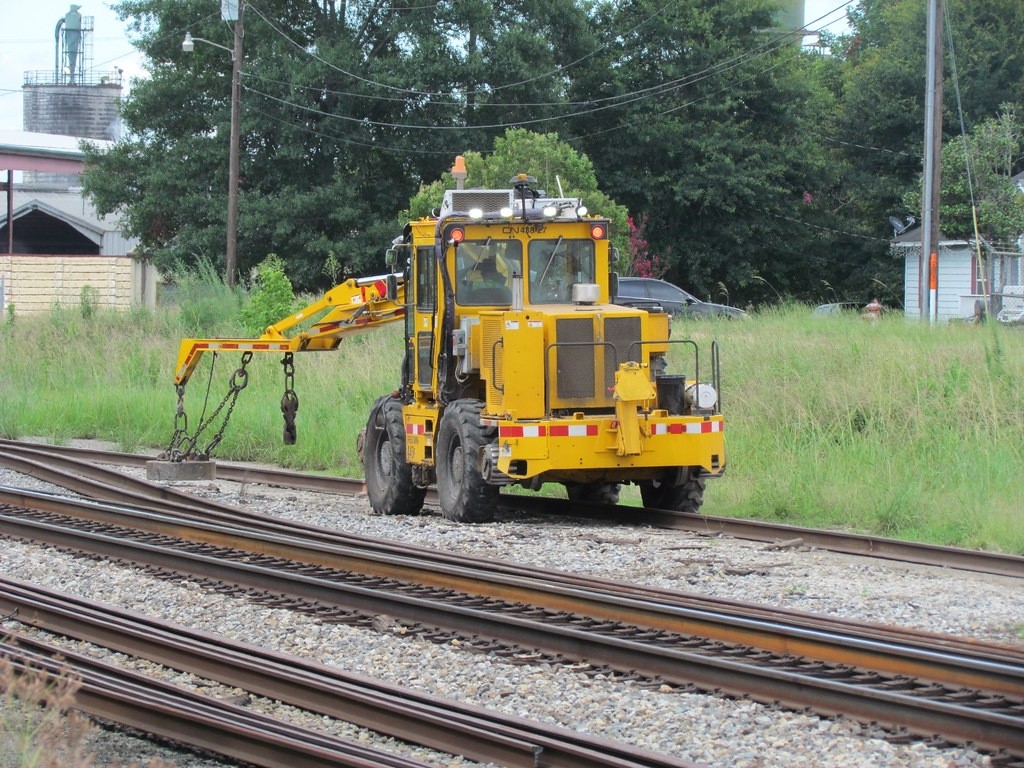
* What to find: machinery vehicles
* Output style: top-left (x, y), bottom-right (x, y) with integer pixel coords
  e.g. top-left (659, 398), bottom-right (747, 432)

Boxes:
top-left (158, 156), bottom-right (726, 520)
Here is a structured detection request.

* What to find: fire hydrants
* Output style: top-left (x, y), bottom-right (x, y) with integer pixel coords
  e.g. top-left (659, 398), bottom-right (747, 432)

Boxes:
top-left (864, 299), bottom-right (882, 321)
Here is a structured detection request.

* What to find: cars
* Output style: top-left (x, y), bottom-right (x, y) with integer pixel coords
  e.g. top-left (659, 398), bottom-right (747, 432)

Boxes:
top-left (811, 300), bottom-right (867, 318)
top-left (611, 277), bottom-right (748, 322)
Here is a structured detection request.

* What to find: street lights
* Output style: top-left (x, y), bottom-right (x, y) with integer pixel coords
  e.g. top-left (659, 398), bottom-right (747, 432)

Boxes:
top-left (183, 31), bottom-right (242, 288)
